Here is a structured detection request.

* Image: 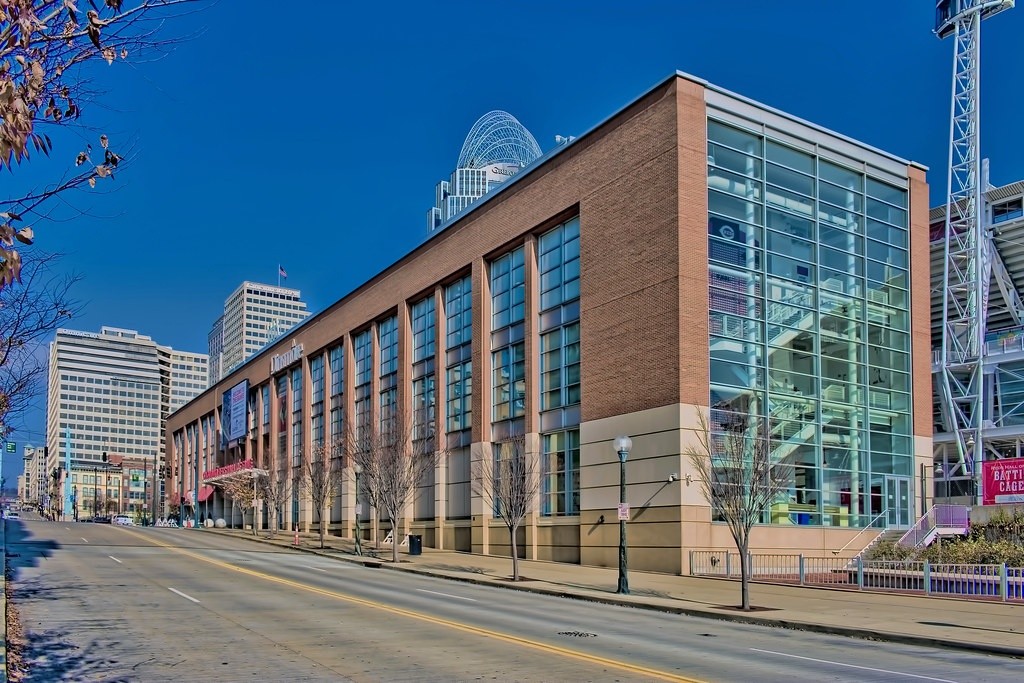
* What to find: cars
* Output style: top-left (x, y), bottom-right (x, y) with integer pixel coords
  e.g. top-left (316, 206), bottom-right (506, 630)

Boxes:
top-left (9, 512), bottom-right (20, 520)
top-left (81, 515), bottom-right (134, 525)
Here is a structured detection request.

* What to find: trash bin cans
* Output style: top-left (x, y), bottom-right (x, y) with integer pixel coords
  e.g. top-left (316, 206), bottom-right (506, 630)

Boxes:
top-left (409, 535), bottom-right (422, 555)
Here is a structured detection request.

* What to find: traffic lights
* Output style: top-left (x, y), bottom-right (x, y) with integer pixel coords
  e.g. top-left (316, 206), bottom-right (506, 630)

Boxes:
top-left (108, 474), bottom-right (110, 480)
top-left (66, 473), bottom-right (68, 477)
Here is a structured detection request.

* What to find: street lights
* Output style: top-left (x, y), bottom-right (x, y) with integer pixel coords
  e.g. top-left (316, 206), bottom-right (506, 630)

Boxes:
top-left (924, 463), bottom-right (943, 529)
top-left (613, 433), bottom-right (632, 594)
top-left (353, 464), bottom-right (366, 556)
